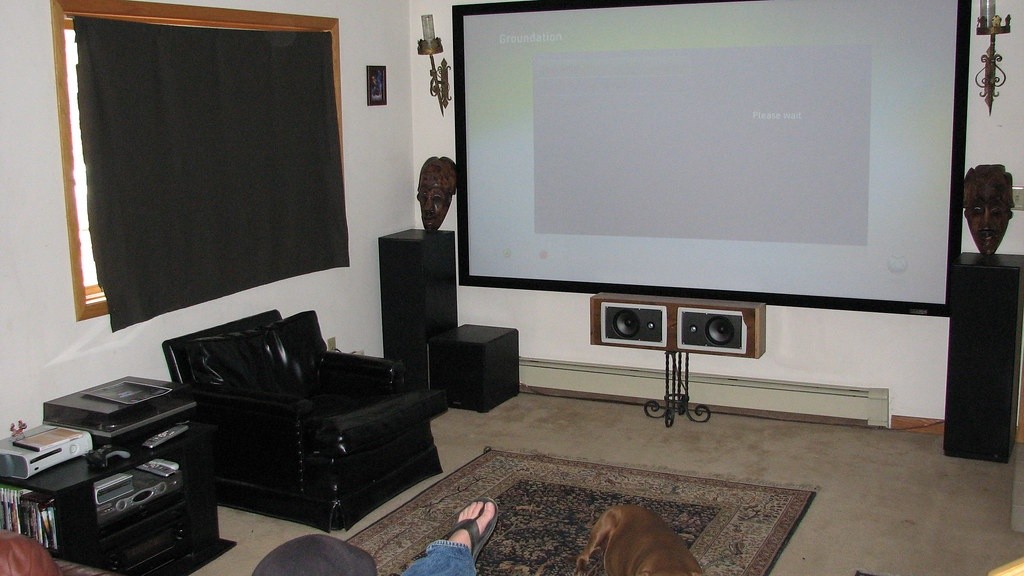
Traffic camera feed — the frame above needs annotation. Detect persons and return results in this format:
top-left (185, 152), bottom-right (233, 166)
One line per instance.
top-left (401, 496), bottom-right (498, 576)
top-left (416, 157), bottom-right (457, 233)
top-left (962, 164), bottom-right (1015, 256)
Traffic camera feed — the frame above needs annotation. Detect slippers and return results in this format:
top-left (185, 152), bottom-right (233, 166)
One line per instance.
top-left (446, 497), bottom-right (498, 563)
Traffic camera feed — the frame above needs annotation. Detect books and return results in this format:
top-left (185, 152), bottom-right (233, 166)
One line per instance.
top-left (0, 488), bottom-right (58, 551)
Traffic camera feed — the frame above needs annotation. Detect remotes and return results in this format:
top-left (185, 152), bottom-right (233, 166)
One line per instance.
top-left (142, 424), bottom-right (189, 450)
top-left (136, 459), bottom-right (175, 477)
top-left (148, 458), bottom-right (179, 470)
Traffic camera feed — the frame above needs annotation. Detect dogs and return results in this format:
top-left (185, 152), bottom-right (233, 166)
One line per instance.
top-left (575, 504), bottom-right (704, 576)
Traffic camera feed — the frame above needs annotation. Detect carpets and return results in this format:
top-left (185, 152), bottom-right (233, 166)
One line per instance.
top-left (344, 446), bottom-right (822, 576)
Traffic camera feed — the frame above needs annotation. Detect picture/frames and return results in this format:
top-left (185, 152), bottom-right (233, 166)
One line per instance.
top-left (366, 65), bottom-right (387, 106)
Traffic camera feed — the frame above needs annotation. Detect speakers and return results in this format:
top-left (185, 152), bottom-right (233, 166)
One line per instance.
top-left (589, 293), bottom-right (767, 359)
top-left (426, 325), bottom-right (520, 413)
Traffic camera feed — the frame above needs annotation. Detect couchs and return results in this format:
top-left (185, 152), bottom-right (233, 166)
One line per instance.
top-left (162, 309), bottom-right (446, 534)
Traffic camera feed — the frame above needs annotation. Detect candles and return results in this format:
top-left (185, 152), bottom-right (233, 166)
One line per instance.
top-left (979, 0), bottom-right (995, 28)
top-left (422, 14), bottom-right (435, 47)
